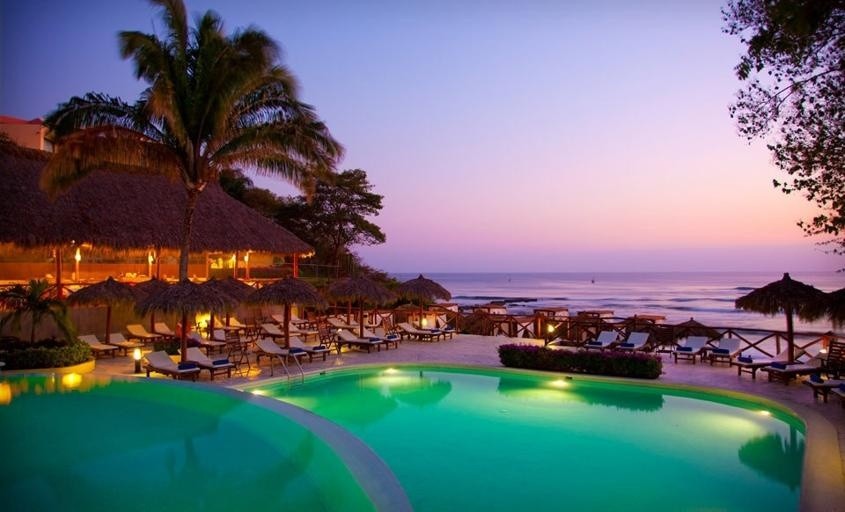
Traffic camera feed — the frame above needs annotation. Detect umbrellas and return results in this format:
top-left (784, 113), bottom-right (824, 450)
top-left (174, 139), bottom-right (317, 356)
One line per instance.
top-left (655, 317), bottom-right (723, 344)
top-left (735, 272), bottom-right (830, 363)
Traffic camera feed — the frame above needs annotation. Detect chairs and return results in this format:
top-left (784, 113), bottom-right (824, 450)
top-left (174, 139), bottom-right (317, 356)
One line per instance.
top-left (581, 326), bottom-right (845, 411)
top-left (75, 308), bottom-right (456, 382)
